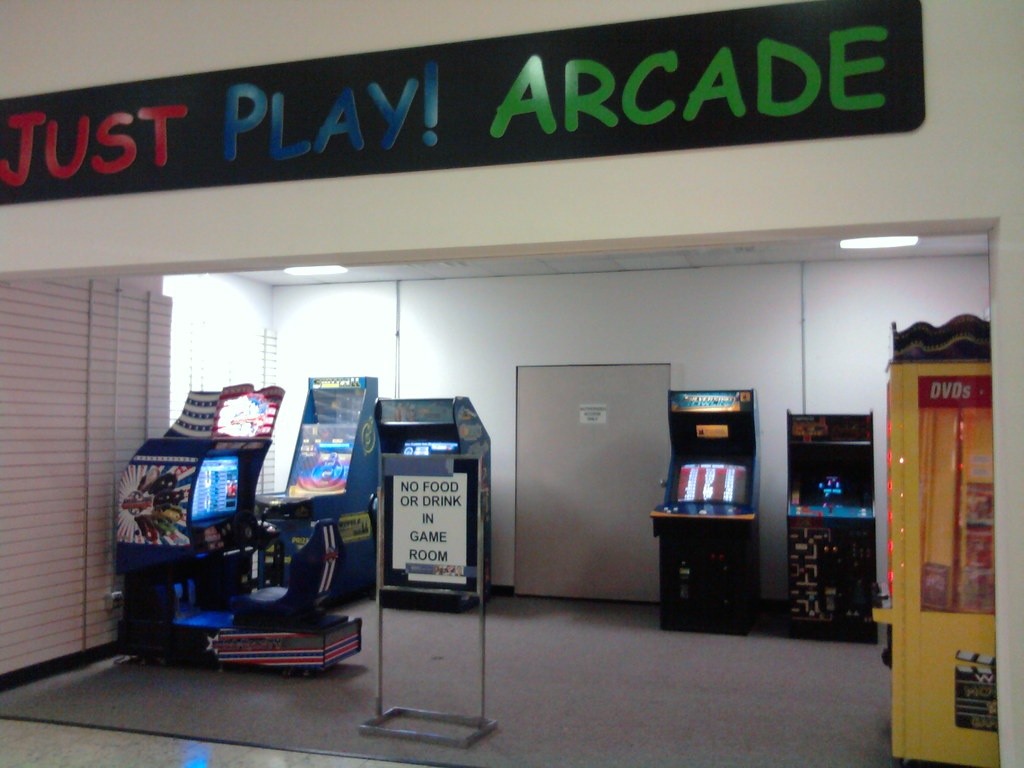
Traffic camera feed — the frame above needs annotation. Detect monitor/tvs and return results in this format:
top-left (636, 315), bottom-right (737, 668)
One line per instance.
top-left (190, 456), bottom-right (239, 521)
top-left (675, 461), bottom-right (747, 507)
top-left (800, 464), bottom-right (861, 507)
top-left (401, 438), bottom-right (460, 455)
